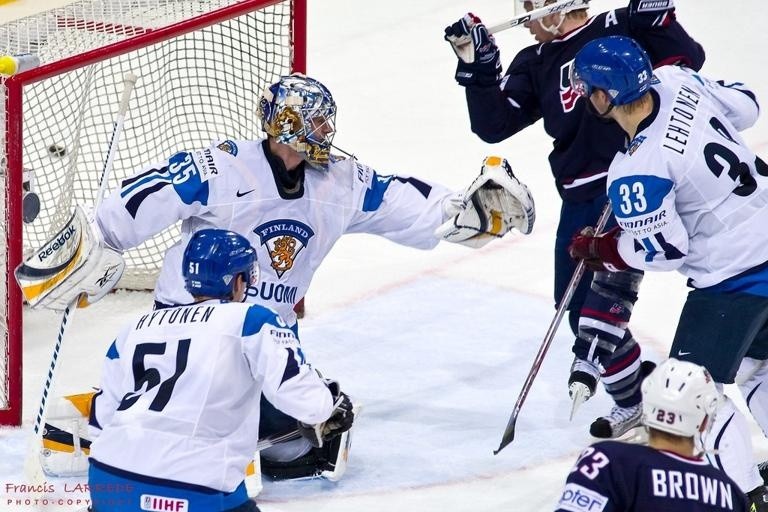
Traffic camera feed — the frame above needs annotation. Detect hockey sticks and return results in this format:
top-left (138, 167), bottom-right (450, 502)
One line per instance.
top-left (22, 73), bottom-right (139, 509)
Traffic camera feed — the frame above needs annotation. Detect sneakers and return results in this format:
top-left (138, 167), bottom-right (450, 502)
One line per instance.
top-left (570, 357), bottom-right (599, 397)
top-left (744, 462), bottom-right (767, 512)
top-left (591, 403), bottom-right (644, 440)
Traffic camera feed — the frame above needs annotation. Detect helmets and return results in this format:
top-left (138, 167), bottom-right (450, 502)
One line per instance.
top-left (257, 72), bottom-right (335, 174)
top-left (568, 35), bottom-right (653, 105)
top-left (181, 227), bottom-right (260, 300)
top-left (513, 0), bottom-right (591, 17)
top-left (641, 357), bottom-right (719, 451)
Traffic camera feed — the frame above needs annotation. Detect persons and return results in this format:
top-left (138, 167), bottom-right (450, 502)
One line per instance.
top-left (12, 72), bottom-right (535, 479)
top-left (548, 359), bottom-right (750, 512)
top-left (570, 35), bottom-right (768, 388)
top-left (443, 0), bottom-right (706, 440)
top-left (36, 230), bottom-right (353, 512)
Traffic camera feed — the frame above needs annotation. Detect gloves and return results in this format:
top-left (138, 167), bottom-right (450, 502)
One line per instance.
top-left (322, 391), bottom-right (354, 441)
top-left (444, 11), bottom-right (505, 86)
top-left (569, 227), bottom-right (644, 278)
